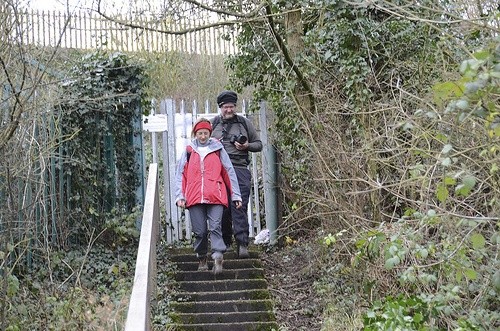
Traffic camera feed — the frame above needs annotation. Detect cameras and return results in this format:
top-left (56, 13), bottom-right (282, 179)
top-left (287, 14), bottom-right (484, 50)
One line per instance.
top-left (230, 134), bottom-right (247, 144)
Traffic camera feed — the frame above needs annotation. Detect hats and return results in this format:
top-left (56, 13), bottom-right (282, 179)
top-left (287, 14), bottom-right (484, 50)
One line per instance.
top-left (216, 91), bottom-right (238, 108)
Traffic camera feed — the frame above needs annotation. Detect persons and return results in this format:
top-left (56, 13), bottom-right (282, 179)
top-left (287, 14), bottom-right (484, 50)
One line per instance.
top-left (208, 89), bottom-right (262, 258)
top-left (177, 117), bottom-right (241, 275)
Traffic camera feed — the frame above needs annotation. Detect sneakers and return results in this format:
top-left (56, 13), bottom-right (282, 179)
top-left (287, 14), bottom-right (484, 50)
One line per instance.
top-left (212, 258), bottom-right (225, 275)
top-left (237, 243), bottom-right (249, 258)
top-left (197, 256), bottom-right (209, 271)
top-left (225, 244), bottom-right (234, 252)
